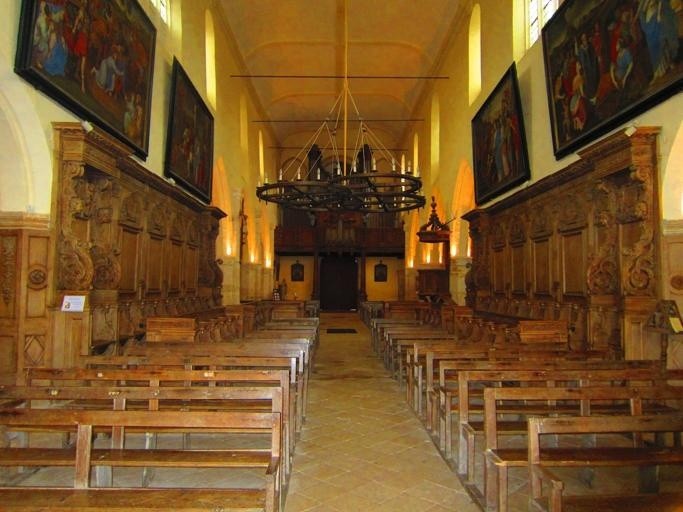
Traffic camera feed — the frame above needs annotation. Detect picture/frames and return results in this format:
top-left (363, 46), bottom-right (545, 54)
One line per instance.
top-left (163, 53), bottom-right (214, 205)
top-left (539, 0), bottom-right (682, 163)
top-left (469, 60), bottom-right (530, 206)
top-left (12, 0), bottom-right (157, 162)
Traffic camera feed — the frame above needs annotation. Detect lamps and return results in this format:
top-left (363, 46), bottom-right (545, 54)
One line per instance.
top-left (641, 299), bottom-right (682, 382)
top-left (255, 0), bottom-right (425, 210)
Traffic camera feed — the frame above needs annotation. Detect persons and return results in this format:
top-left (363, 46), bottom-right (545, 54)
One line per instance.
top-left (33, 2), bottom-right (149, 147)
top-left (553, 0), bottom-right (683, 140)
top-left (471, 99), bottom-right (526, 198)
